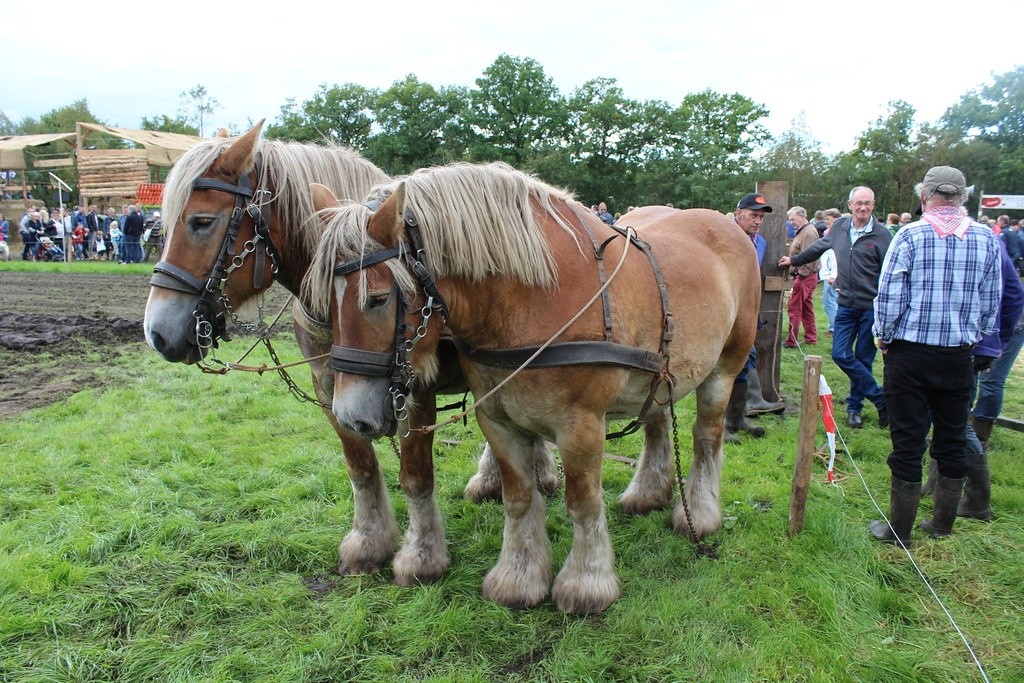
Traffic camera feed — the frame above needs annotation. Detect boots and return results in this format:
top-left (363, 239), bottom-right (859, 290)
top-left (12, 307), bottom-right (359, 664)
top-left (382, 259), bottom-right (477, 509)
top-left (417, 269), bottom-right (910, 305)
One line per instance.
top-left (723, 428), bottom-right (741, 445)
top-left (956, 452), bottom-right (992, 519)
top-left (870, 475), bottom-right (922, 550)
top-left (920, 458), bottom-right (938, 496)
top-left (724, 381), bottom-right (766, 438)
top-left (970, 414), bottom-right (993, 449)
top-left (918, 473), bottom-right (965, 539)
top-left (744, 369), bottom-right (785, 418)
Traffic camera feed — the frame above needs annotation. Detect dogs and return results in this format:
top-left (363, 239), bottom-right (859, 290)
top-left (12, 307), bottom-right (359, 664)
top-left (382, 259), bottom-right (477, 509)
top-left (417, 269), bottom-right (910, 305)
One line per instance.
top-left (0, 241), bottom-right (10, 263)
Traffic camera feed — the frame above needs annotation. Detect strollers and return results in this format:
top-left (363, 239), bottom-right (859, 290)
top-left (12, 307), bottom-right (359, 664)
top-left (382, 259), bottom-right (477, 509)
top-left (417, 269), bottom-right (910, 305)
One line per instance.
top-left (35, 232), bottom-right (64, 261)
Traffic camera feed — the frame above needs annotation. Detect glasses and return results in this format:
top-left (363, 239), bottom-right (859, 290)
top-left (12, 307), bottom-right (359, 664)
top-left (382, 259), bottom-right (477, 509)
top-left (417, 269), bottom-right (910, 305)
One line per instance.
top-left (852, 202), bottom-right (874, 207)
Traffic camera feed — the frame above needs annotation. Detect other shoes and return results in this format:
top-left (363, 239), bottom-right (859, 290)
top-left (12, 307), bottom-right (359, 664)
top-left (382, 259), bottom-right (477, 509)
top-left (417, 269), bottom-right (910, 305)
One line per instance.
top-left (783, 344), bottom-right (793, 349)
top-left (799, 341), bottom-right (810, 347)
top-left (824, 330), bottom-right (834, 338)
top-left (75, 254), bottom-right (113, 261)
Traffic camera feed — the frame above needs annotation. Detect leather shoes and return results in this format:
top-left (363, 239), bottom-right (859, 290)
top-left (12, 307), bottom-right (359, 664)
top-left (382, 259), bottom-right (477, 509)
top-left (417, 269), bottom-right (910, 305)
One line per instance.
top-left (878, 409), bottom-right (889, 429)
top-left (848, 412), bottom-right (862, 428)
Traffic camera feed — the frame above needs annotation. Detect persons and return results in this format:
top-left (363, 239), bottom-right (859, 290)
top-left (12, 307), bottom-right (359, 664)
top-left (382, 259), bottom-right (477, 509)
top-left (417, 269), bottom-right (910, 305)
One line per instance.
top-left (721, 192), bottom-right (851, 444)
top-left (0, 179), bottom-right (166, 265)
top-left (591, 201), bottom-right (673, 224)
top-left (776, 185), bottom-right (888, 429)
top-left (871, 166), bottom-right (1002, 547)
top-left (879, 213), bottom-right (1024, 520)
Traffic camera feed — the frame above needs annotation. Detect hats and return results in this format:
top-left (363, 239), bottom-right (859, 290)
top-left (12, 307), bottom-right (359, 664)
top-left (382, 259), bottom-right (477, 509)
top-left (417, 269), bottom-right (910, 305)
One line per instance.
top-left (737, 193), bottom-right (773, 213)
top-left (153, 211), bottom-right (161, 218)
top-left (923, 165), bottom-right (966, 195)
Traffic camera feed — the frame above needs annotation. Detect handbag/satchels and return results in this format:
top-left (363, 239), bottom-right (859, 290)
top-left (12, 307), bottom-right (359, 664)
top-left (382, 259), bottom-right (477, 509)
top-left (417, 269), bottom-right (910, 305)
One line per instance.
top-left (143, 229), bottom-right (152, 242)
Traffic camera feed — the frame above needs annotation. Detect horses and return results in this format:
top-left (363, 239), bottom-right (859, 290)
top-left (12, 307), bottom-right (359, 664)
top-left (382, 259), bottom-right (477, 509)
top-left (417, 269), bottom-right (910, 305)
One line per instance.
top-left (300, 160), bottom-right (763, 616)
top-left (143, 118), bottom-right (559, 591)
